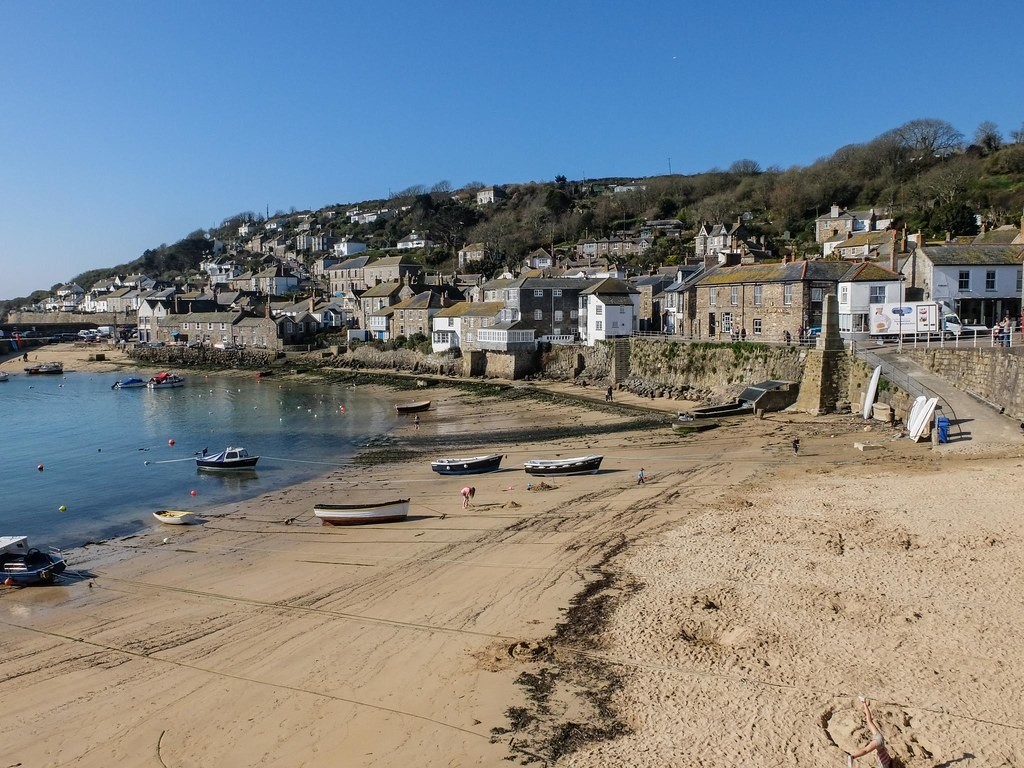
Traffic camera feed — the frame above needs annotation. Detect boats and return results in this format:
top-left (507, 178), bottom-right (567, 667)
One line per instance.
top-left (0, 372), bottom-right (9, 382)
top-left (524, 455), bottom-right (606, 477)
top-left (395, 400), bottom-right (432, 413)
top-left (907, 396), bottom-right (927, 432)
top-left (0, 535), bottom-right (67, 586)
top-left (112, 378), bottom-right (148, 389)
top-left (313, 498), bottom-right (412, 525)
top-left (863, 365), bottom-right (882, 419)
top-left (154, 509), bottom-right (195, 525)
top-left (196, 447), bottom-right (260, 470)
top-left (25, 361), bottom-right (64, 374)
top-left (431, 454), bottom-right (504, 476)
top-left (910, 396), bottom-right (940, 443)
top-left (147, 373), bottom-right (184, 389)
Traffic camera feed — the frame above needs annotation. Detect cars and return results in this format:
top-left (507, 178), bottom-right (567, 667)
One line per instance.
top-left (0, 329), bottom-right (109, 339)
top-left (806, 327), bottom-right (821, 347)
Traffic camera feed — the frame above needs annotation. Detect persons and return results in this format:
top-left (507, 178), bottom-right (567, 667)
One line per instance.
top-left (874, 305), bottom-right (892, 333)
top-left (637, 467), bottom-right (645, 485)
top-left (606, 385), bottom-right (613, 402)
top-left (460, 486), bottom-right (475, 508)
top-left (793, 438), bottom-right (800, 455)
top-left (993, 315), bottom-right (1011, 347)
top-left (729, 326), bottom-right (746, 344)
top-left (797, 325), bottom-right (804, 346)
top-left (661, 324), bottom-right (669, 341)
top-left (414, 413), bottom-right (420, 430)
top-left (783, 330), bottom-right (790, 346)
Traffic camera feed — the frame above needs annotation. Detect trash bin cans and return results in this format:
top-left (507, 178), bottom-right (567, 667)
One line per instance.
top-left (938, 417), bottom-right (949, 423)
top-left (938, 422), bottom-right (948, 442)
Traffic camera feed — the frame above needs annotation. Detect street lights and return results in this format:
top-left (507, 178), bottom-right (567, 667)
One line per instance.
top-left (898, 270), bottom-right (905, 348)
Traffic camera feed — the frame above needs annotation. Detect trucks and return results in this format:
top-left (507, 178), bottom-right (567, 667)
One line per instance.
top-left (869, 301), bottom-right (963, 344)
top-left (97, 326), bottom-right (115, 339)
